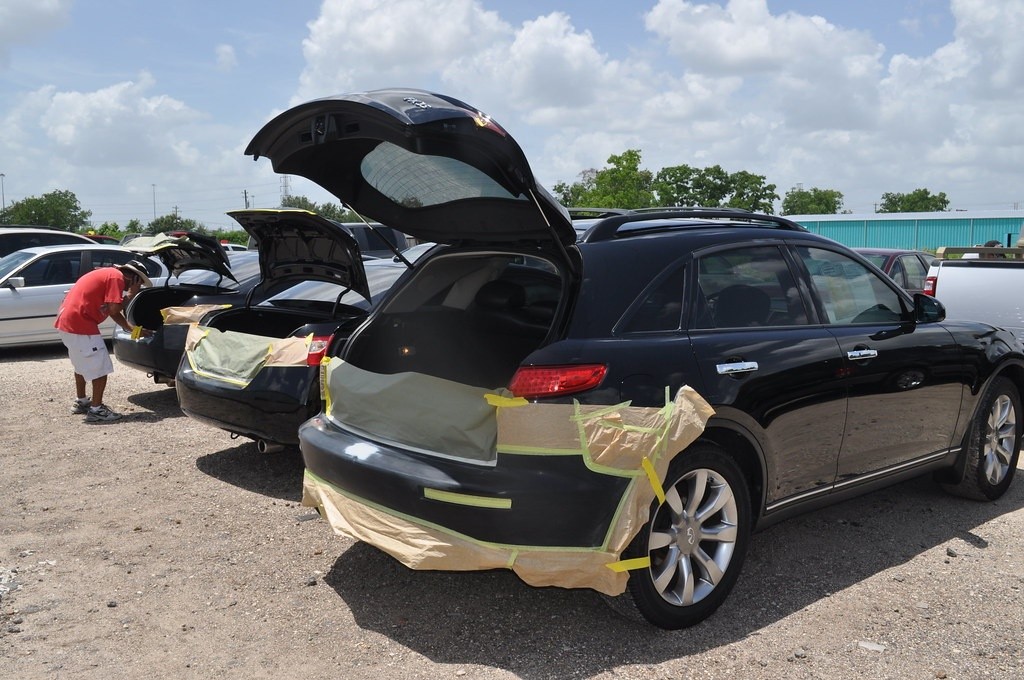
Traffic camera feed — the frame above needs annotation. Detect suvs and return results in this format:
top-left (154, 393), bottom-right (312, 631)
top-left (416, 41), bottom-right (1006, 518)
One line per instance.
top-left (0, 224), bottom-right (101, 282)
top-left (243, 87), bottom-right (1023, 629)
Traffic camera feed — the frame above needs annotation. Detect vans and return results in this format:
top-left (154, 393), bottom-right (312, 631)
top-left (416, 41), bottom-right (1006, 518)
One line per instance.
top-left (247, 223), bottom-right (409, 259)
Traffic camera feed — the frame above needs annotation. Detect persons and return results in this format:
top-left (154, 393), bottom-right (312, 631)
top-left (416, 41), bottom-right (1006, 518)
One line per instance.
top-left (54, 260), bottom-right (155, 421)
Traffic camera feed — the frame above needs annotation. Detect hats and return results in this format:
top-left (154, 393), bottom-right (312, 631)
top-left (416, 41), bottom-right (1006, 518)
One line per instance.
top-left (114, 259), bottom-right (153, 286)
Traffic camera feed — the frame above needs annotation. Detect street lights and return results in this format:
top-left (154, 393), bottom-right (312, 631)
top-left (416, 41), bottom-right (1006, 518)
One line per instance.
top-left (152, 184), bottom-right (156, 219)
top-left (0, 174), bottom-right (5, 210)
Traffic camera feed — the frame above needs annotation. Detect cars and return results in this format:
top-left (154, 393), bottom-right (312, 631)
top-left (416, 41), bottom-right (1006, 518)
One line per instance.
top-left (0, 244), bottom-right (176, 348)
top-left (174, 207), bottom-right (437, 454)
top-left (731, 247), bottom-right (937, 325)
top-left (112, 229), bottom-right (261, 387)
top-left (699, 255), bottom-right (764, 297)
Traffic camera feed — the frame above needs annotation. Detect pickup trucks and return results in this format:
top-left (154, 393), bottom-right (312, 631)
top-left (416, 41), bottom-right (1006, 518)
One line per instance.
top-left (923, 247), bottom-right (1024, 343)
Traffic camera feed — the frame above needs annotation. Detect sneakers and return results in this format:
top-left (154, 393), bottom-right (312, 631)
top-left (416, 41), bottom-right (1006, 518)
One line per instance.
top-left (71, 400), bottom-right (93, 414)
top-left (87, 404), bottom-right (122, 422)
